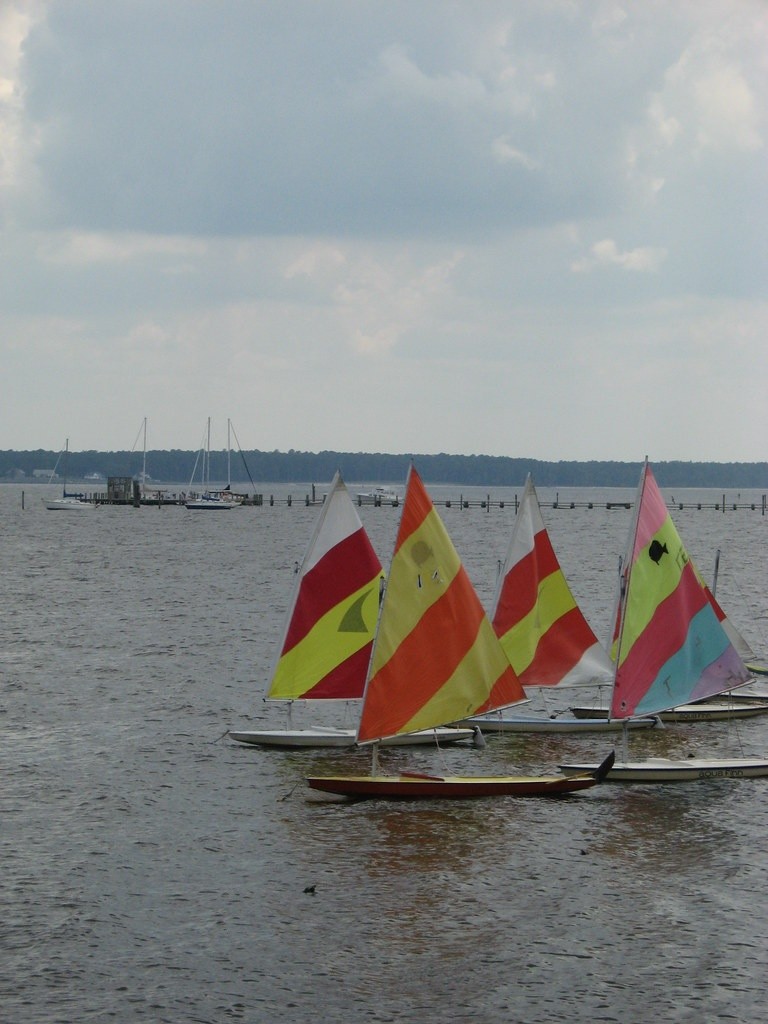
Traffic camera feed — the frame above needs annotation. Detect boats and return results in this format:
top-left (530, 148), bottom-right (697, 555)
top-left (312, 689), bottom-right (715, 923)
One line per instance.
top-left (355, 486), bottom-right (406, 503)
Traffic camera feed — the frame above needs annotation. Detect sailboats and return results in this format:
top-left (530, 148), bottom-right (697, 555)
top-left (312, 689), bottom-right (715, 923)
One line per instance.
top-left (555, 451), bottom-right (767, 782)
top-left (40, 438), bottom-right (102, 510)
top-left (183, 416), bottom-right (257, 510)
top-left (302, 456), bottom-right (616, 801)
top-left (123, 417), bottom-right (155, 500)
top-left (226, 468), bottom-right (488, 747)
top-left (441, 468), bottom-right (658, 732)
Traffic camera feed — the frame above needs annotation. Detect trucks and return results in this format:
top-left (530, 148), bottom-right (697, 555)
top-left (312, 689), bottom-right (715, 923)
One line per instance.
top-left (33, 469), bottom-right (59, 478)
